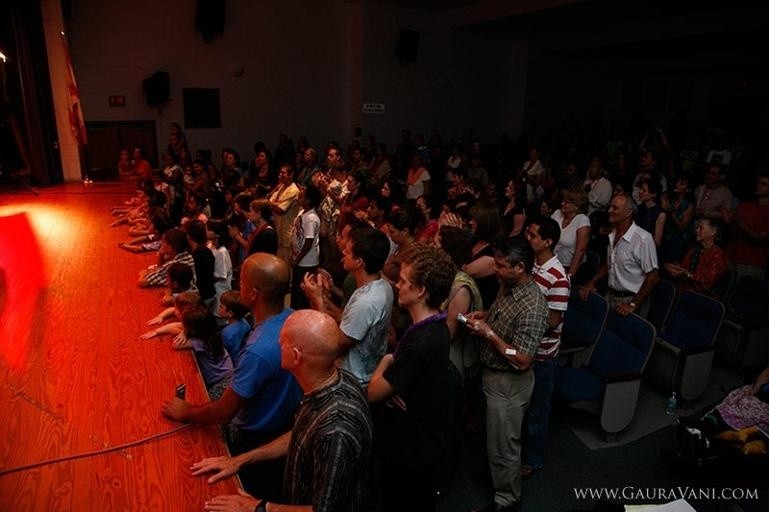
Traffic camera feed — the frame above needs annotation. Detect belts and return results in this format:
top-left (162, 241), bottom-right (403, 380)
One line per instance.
top-left (607, 286), bottom-right (641, 298)
top-left (482, 364), bottom-right (533, 375)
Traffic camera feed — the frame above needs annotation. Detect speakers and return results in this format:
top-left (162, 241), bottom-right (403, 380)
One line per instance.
top-left (396, 28), bottom-right (420, 63)
top-left (195, 0), bottom-right (226, 41)
top-left (141, 70), bottom-right (169, 105)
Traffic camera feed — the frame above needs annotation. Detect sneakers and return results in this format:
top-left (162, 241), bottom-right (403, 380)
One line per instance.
top-left (519, 461), bottom-right (547, 474)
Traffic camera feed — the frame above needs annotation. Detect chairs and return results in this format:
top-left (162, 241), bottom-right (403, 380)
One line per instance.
top-left (723, 274), bottom-right (764, 329)
top-left (706, 266), bottom-right (734, 305)
top-left (637, 281), bottom-right (677, 334)
top-left (550, 307), bottom-right (657, 405)
top-left (557, 282), bottom-right (610, 354)
top-left (654, 289), bottom-right (726, 399)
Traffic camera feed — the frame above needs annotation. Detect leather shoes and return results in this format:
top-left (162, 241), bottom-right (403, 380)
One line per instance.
top-left (479, 496), bottom-right (524, 512)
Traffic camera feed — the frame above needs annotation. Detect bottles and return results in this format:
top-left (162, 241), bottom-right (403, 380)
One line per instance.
top-left (666, 390), bottom-right (678, 417)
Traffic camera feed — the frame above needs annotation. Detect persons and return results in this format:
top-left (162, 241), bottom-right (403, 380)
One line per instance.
top-left (110, 122), bottom-right (768, 511)
